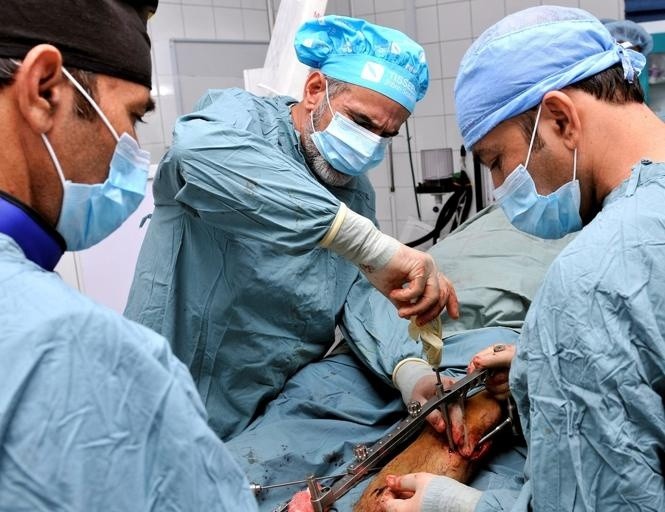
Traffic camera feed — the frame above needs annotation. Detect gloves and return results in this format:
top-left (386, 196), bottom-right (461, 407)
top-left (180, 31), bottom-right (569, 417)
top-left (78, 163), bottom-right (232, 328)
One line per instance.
top-left (468, 343), bottom-right (516, 401)
top-left (392, 357), bottom-right (475, 457)
top-left (380, 472), bottom-right (483, 512)
top-left (318, 200), bottom-right (460, 327)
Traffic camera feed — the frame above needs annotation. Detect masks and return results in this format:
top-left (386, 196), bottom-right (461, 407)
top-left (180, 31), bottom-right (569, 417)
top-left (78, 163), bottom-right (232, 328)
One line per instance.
top-left (311, 79), bottom-right (393, 177)
top-left (491, 104), bottom-right (593, 240)
top-left (41, 65), bottom-right (152, 251)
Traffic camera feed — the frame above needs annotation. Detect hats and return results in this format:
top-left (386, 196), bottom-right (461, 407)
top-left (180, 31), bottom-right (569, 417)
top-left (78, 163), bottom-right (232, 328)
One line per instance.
top-left (1, 0), bottom-right (157, 90)
top-left (293, 15), bottom-right (429, 114)
top-left (454, 4), bottom-right (647, 151)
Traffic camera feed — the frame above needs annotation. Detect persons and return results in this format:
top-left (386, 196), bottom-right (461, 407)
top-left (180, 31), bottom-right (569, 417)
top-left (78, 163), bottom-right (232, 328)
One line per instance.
top-left (122, 13), bottom-right (460, 442)
top-left (380, 5), bottom-right (665, 512)
top-left (605, 18), bottom-right (652, 104)
top-left (0, 0), bottom-right (260, 512)
top-left (351, 388), bottom-right (501, 512)
